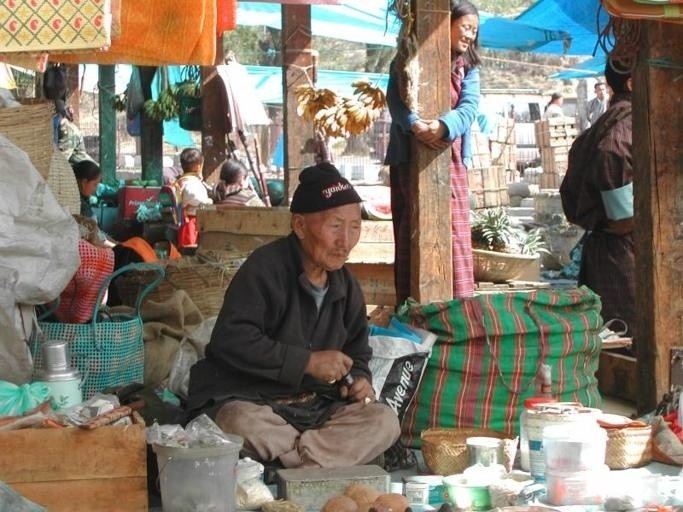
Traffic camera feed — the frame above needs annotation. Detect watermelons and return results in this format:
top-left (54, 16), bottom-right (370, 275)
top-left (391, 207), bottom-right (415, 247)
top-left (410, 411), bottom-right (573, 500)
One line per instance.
top-left (249, 176), bottom-right (285, 206)
top-left (361, 199), bottom-right (393, 221)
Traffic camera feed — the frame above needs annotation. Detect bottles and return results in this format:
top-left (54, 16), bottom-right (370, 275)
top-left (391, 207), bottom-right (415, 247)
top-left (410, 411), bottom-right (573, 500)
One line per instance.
top-left (519, 396), bottom-right (557, 472)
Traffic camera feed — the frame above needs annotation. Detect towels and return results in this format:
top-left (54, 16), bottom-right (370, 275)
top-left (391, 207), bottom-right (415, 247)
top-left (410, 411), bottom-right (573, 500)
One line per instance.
top-left (0, 0), bottom-right (235, 74)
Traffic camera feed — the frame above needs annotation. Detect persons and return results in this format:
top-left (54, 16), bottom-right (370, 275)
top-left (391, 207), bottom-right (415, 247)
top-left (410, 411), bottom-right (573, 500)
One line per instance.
top-left (161, 147), bottom-right (212, 249)
top-left (560, 48), bottom-right (637, 358)
top-left (586, 82), bottom-right (611, 130)
top-left (382, 0), bottom-right (482, 303)
top-left (52, 98), bottom-right (92, 164)
top-left (208, 160), bottom-right (267, 208)
top-left (542, 92), bottom-right (564, 118)
top-left (76, 157), bottom-right (119, 251)
top-left (185, 160), bottom-right (403, 485)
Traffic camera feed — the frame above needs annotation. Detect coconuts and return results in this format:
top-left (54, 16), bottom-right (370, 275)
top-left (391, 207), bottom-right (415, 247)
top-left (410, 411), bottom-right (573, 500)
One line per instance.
top-left (321, 486), bottom-right (409, 512)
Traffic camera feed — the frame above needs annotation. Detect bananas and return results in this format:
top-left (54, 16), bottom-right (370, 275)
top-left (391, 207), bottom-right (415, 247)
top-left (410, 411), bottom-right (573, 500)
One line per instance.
top-left (143, 79), bottom-right (200, 123)
top-left (294, 79), bottom-right (387, 140)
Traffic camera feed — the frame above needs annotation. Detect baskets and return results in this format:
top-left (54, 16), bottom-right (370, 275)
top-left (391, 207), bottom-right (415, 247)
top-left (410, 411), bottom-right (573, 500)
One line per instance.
top-left (472, 249), bottom-right (540, 282)
top-left (421, 427), bottom-right (519, 477)
top-left (605, 426), bottom-right (653, 470)
top-left (0, 100), bottom-right (81, 216)
top-left (114, 249), bottom-right (253, 318)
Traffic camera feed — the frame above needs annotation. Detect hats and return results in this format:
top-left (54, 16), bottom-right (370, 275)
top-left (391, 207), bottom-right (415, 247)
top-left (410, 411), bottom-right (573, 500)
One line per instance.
top-left (290, 161), bottom-right (363, 213)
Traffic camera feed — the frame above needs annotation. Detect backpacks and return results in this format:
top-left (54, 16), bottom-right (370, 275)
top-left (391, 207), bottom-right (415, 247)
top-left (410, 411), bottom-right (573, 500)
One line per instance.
top-left (158, 174), bottom-right (196, 230)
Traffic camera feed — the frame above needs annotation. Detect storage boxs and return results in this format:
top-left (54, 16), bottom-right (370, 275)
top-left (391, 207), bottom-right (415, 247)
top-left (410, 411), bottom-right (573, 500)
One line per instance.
top-left (0, 411), bottom-right (149, 512)
top-left (342, 221), bottom-right (395, 306)
top-left (195, 207), bottom-right (292, 251)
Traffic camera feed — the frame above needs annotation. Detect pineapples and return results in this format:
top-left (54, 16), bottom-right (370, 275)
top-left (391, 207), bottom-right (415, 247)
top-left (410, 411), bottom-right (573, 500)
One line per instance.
top-left (468, 206), bottom-right (553, 273)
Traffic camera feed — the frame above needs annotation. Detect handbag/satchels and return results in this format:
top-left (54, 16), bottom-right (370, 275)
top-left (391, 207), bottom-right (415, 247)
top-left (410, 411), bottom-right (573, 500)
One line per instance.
top-left (368, 285), bottom-right (604, 450)
top-left (31, 238), bottom-right (165, 402)
top-left (179, 83), bottom-right (203, 131)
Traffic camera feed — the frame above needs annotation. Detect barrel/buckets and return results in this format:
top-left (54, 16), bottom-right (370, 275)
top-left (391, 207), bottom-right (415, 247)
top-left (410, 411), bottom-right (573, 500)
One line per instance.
top-left (524, 408), bottom-right (603, 485)
top-left (151, 431), bottom-right (245, 512)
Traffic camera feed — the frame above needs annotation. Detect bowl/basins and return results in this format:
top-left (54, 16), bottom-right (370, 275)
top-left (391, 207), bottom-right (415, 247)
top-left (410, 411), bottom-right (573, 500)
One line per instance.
top-left (443, 473), bottom-right (494, 511)
